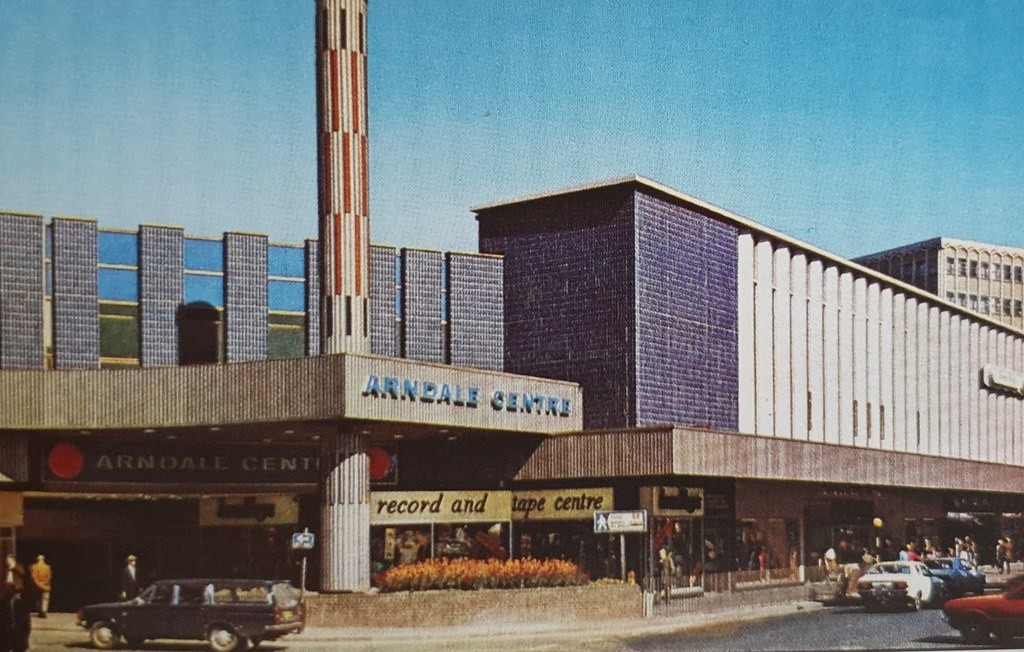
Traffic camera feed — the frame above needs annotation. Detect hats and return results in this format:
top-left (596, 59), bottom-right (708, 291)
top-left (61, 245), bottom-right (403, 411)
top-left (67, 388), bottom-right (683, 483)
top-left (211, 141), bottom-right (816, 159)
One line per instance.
top-left (127, 554), bottom-right (137, 560)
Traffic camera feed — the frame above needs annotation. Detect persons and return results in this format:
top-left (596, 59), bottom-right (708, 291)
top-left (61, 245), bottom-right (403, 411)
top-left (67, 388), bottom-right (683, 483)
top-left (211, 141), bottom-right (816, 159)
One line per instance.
top-left (120, 555), bottom-right (148, 600)
top-left (1, 584), bottom-right (30, 652)
top-left (882, 535), bottom-right (1013, 576)
top-left (2, 554), bottom-right (25, 595)
top-left (30, 553), bottom-right (54, 618)
top-left (157, 586), bottom-right (170, 601)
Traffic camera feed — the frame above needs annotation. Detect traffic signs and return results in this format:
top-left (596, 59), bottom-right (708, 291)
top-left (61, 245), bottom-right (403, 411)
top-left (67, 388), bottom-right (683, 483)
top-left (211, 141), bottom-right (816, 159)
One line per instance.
top-left (292, 534), bottom-right (315, 548)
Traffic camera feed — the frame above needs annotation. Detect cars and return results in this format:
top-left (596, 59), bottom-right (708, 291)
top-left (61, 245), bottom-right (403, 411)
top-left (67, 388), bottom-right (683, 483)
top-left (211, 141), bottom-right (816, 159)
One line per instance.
top-left (857, 561), bottom-right (948, 612)
top-left (941, 573), bottom-right (1024, 644)
top-left (75, 576), bottom-right (305, 652)
top-left (928, 557), bottom-right (986, 596)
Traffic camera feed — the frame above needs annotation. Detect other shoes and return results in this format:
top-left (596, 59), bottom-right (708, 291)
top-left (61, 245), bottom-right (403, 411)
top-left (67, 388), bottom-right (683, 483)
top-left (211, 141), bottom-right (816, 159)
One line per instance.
top-left (846, 590), bottom-right (862, 598)
top-left (38, 611), bottom-right (47, 617)
